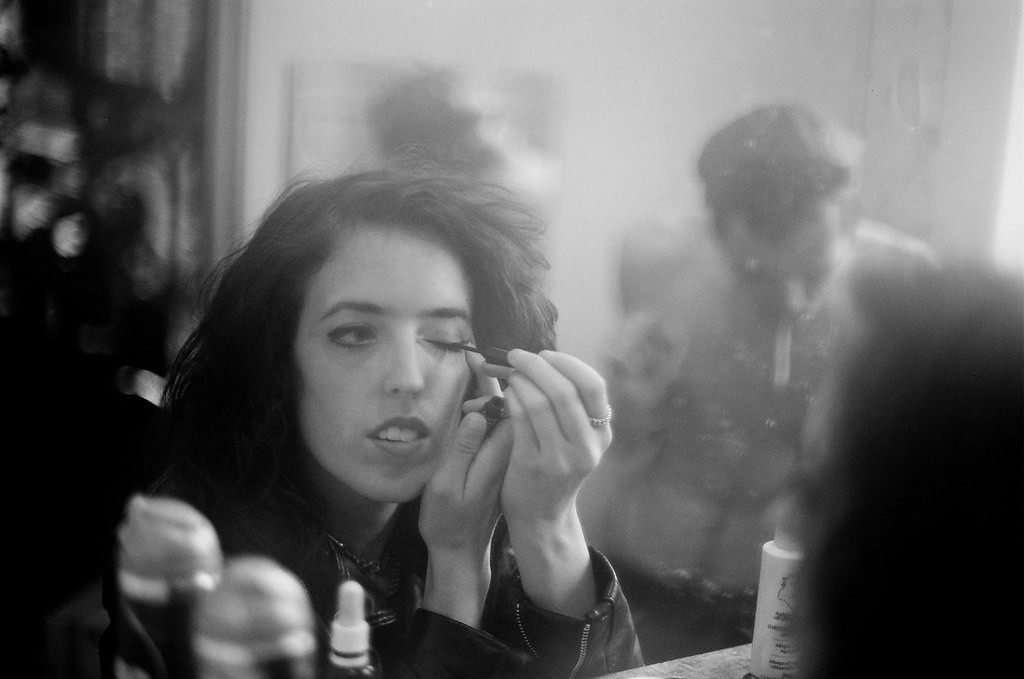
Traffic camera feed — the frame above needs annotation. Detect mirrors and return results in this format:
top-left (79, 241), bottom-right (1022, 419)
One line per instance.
top-left (240, 4), bottom-right (1010, 593)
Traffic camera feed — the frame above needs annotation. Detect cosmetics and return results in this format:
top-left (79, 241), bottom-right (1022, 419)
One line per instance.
top-left (445, 342), bottom-right (518, 436)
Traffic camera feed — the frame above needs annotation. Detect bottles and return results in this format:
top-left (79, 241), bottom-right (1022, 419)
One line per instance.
top-left (748, 507), bottom-right (810, 678)
top-left (328, 580), bottom-right (382, 679)
top-left (193, 557), bottom-right (315, 678)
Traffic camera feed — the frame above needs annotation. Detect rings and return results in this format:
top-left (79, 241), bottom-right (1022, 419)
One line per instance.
top-left (586, 404), bottom-right (613, 427)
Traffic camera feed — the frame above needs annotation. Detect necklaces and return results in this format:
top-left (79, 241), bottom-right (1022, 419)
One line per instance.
top-left (326, 529), bottom-right (399, 630)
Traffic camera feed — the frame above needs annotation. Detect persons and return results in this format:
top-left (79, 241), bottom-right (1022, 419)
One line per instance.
top-left (657, 94), bottom-right (944, 542)
top-left (100, 140), bottom-right (647, 679)
top-left (774, 260), bottom-right (1024, 679)
